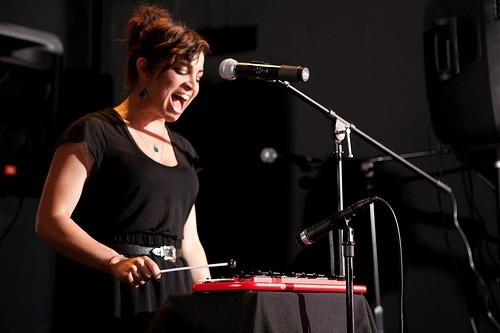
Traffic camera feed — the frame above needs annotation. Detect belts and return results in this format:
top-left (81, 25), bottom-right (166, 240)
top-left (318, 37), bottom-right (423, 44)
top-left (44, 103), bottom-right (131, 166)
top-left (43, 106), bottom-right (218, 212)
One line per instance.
top-left (103, 243), bottom-right (183, 264)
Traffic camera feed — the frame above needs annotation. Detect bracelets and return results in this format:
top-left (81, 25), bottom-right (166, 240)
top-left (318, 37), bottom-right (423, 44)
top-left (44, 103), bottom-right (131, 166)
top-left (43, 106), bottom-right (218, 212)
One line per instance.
top-left (107, 254), bottom-right (124, 274)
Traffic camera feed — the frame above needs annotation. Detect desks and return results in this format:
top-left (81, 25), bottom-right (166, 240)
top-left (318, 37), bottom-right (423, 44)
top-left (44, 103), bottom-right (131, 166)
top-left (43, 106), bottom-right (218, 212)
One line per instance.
top-left (148, 291), bottom-right (378, 333)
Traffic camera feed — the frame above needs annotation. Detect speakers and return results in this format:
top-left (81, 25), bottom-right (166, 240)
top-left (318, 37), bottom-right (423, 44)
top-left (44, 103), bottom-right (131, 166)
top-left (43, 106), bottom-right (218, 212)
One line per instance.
top-left (1, 58), bottom-right (116, 198)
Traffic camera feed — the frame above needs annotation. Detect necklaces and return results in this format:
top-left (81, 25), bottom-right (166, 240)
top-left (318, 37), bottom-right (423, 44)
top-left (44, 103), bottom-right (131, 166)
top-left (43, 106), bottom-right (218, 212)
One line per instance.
top-left (122, 102), bottom-right (165, 153)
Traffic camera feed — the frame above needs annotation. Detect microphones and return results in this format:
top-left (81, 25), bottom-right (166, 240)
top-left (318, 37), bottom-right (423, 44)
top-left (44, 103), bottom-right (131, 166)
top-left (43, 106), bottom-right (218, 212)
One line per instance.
top-left (219, 58), bottom-right (309, 82)
top-left (296, 198), bottom-right (375, 249)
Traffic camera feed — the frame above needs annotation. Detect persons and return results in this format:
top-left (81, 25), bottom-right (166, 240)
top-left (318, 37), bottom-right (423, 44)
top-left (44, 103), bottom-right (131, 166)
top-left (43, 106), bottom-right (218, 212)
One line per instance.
top-left (34, 6), bottom-right (213, 333)
